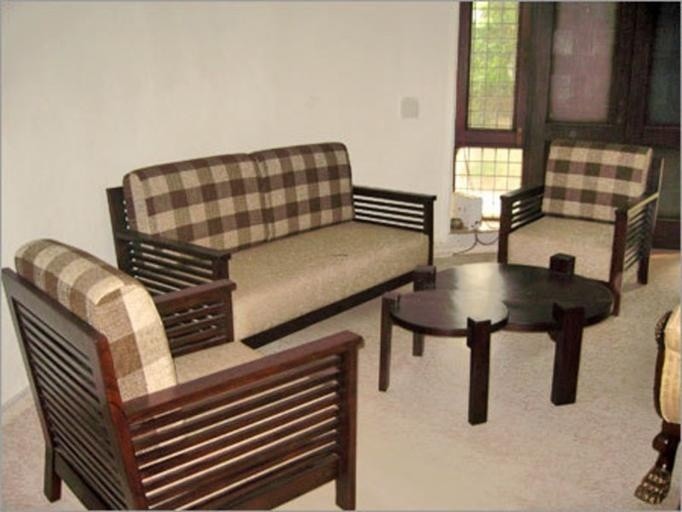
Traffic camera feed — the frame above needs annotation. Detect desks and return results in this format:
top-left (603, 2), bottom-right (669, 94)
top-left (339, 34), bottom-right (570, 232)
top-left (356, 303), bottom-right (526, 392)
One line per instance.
top-left (378, 254), bottom-right (613, 425)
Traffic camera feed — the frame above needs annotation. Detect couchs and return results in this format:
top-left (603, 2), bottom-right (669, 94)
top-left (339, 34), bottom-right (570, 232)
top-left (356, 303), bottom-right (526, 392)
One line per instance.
top-left (107, 143), bottom-right (438, 349)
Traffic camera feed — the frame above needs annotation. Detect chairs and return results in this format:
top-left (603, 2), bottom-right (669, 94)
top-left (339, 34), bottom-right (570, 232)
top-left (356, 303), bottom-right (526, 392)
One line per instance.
top-left (632, 306), bottom-right (682, 504)
top-left (498, 138), bottom-right (665, 317)
top-left (2, 236), bottom-right (365, 511)
